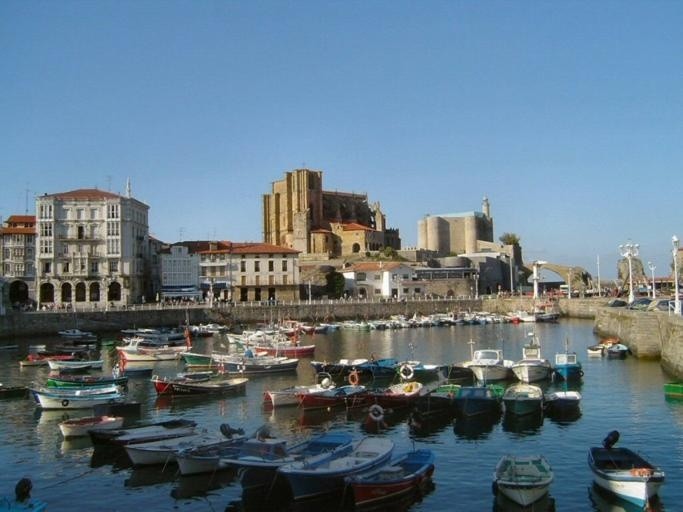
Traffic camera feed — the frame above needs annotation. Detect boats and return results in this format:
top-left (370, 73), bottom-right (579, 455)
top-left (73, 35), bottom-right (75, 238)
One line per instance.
top-left (510, 324), bottom-right (554, 384)
top-left (85, 413), bottom-right (196, 448)
top-left (219, 428), bottom-right (352, 492)
top-left (542, 376), bottom-right (584, 417)
top-left (0, 306), bottom-right (586, 418)
top-left (341, 448), bottom-right (437, 508)
top-left (276, 435), bottom-right (397, 505)
top-left (264, 371), bottom-right (338, 410)
top-left (293, 380), bottom-right (367, 411)
top-left (464, 347), bottom-right (515, 383)
top-left (346, 354), bottom-right (398, 388)
top-left (109, 424), bottom-right (207, 448)
top-left (588, 432), bottom-right (669, 505)
top-left (120, 423), bottom-right (250, 466)
top-left (587, 336), bottom-right (630, 360)
top-left (415, 383), bottom-right (461, 412)
top-left (493, 454), bottom-right (553, 505)
top-left (366, 382), bottom-right (423, 410)
top-left (438, 360), bottom-right (474, 382)
top-left (501, 377), bottom-right (544, 417)
top-left (451, 384), bottom-right (505, 418)
top-left (396, 343), bottom-right (441, 382)
top-left (57, 414), bottom-right (125, 438)
top-left (552, 336), bottom-right (585, 384)
top-left (174, 423), bottom-right (276, 476)
top-left (308, 356), bottom-right (370, 384)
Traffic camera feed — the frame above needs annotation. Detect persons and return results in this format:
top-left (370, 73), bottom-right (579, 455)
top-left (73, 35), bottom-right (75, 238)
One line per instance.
top-left (13, 295), bottom-right (274, 311)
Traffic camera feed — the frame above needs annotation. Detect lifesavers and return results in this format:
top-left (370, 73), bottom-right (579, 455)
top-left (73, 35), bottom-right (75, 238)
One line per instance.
top-left (369, 405), bottom-right (384, 421)
top-left (400, 364), bottom-right (414, 380)
top-left (348, 373), bottom-right (358, 385)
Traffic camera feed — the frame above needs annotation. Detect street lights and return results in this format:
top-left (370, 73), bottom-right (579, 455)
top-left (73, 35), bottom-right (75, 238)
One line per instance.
top-left (618, 242), bottom-right (639, 305)
top-left (647, 261), bottom-right (657, 300)
top-left (670, 235), bottom-right (681, 314)
